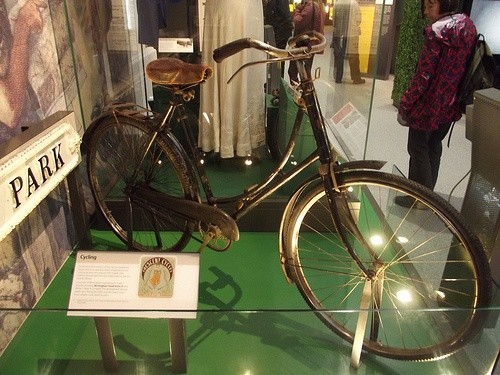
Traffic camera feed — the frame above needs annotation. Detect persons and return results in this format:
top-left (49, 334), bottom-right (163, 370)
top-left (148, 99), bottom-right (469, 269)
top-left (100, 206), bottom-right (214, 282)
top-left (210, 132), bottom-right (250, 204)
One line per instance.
top-left (265, 0), bottom-right (293, 79)
top-left (332, 0), bottom-right (365, 85)
top-left (291, 0), bottom-right (326, 90)
top-left (393, 0), bottom-right (477, 210)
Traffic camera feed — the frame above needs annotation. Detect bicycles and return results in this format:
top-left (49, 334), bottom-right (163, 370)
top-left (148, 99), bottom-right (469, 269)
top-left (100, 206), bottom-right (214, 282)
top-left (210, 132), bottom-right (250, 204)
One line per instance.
top-left (79, 30), bottom-right (493, 371)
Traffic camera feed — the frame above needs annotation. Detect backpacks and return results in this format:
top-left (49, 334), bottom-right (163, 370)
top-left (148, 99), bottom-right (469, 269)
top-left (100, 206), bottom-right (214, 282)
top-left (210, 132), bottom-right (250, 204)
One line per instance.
top-left (456, 33), bottom-right (494, 114)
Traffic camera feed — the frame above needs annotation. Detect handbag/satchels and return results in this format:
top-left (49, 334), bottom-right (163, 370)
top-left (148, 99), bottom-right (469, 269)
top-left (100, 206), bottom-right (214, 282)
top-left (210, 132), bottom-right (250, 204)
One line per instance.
top-left (293, 30), bottom-right (312, 48)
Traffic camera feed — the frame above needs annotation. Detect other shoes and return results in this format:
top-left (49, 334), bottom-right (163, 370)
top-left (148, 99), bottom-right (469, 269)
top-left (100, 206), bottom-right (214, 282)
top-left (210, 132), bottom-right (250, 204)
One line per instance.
top-left (394, 196), bottom-right (429, 210)
top-left (352, 78), bottom-right (366, 84)
top-left (335, 79), bottom-right (342, 83)
